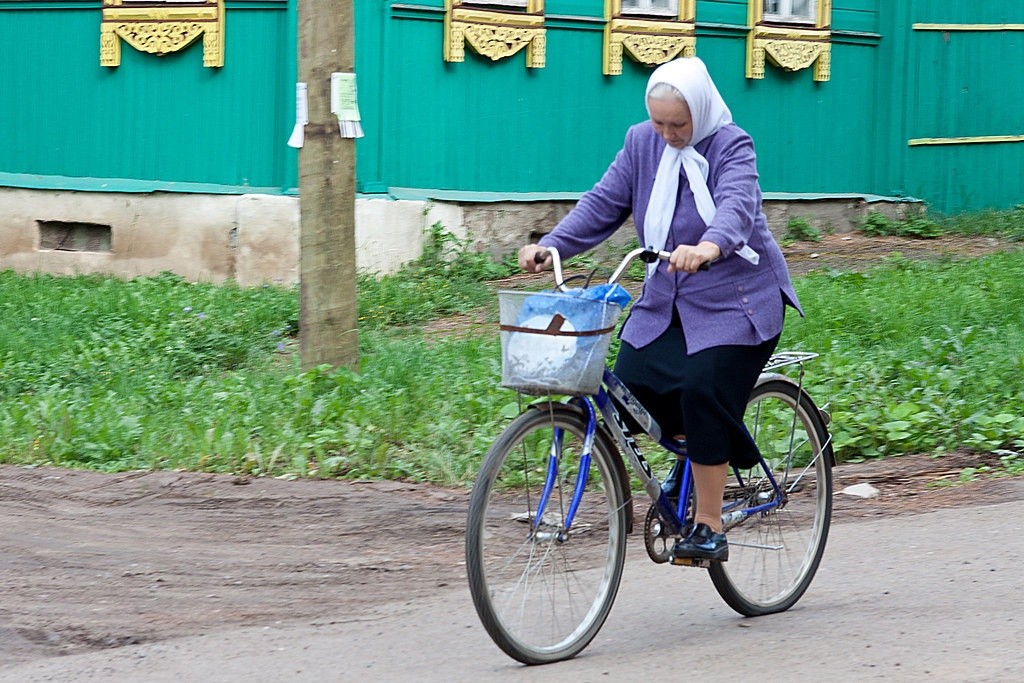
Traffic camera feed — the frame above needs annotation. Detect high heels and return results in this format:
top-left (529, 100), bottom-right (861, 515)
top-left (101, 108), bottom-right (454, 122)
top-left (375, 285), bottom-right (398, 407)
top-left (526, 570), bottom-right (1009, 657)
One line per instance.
top-left (660, 460), bottom-right (687, 497)
top-left (675, 523), bottom-right (729, 561)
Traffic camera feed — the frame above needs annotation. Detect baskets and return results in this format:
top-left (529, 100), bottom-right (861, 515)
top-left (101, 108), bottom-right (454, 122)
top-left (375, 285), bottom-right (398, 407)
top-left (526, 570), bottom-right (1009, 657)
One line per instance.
top-left (496, 288), bottom-right (623, 396)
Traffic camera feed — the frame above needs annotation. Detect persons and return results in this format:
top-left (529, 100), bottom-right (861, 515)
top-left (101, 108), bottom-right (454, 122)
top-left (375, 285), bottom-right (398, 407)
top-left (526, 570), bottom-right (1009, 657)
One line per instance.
top-left (518, 57), bottom-right (804, 561)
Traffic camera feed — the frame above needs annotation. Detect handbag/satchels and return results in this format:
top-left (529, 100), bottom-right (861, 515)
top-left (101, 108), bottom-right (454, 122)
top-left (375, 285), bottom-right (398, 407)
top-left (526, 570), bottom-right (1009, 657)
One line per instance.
top-left (506, 268), bottom-right (633, 396)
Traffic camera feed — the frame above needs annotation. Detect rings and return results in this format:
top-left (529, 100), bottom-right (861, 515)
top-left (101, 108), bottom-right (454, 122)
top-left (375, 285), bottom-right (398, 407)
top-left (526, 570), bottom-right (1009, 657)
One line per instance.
top-left (689, 267), bottom-right (695, 271)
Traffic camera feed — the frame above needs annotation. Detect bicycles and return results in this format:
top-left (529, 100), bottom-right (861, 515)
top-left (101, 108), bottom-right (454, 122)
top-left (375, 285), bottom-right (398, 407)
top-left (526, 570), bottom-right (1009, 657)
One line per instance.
top-left (466, 247), bottom-right (837, 665)
